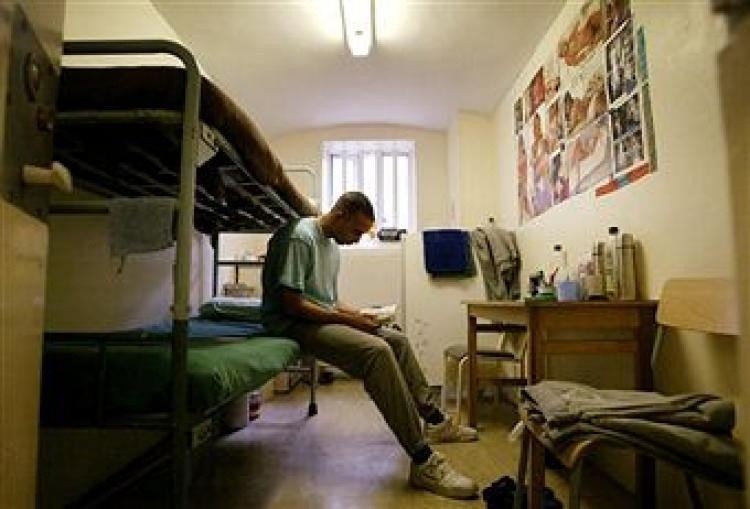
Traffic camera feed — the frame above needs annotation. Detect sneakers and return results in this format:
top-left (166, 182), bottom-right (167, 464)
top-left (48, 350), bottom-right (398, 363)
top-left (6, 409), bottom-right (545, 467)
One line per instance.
top-left (421, 408), bottom-right (477, 443)
top-left (409, 451), bottom-right (480, 499)
top-left (483, 474), bottom-right (563, 509)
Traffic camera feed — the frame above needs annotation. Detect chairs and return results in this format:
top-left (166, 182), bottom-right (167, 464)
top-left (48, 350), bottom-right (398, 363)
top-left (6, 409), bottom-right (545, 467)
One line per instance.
top-left (440, 299), bottom-right (529, 425)
top-left (513, 272), bottom-right (748, 508)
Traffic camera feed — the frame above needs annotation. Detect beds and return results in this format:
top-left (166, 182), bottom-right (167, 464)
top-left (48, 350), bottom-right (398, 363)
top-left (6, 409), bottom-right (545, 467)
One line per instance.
top-left (37, 295), bottom-right (320, 509)
top-left (36, 37), bottom-right (323, 316)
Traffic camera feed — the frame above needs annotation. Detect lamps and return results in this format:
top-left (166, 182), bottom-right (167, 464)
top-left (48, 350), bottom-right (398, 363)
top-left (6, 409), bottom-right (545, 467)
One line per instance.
top-left (339, 0), bottom-right (376, 58)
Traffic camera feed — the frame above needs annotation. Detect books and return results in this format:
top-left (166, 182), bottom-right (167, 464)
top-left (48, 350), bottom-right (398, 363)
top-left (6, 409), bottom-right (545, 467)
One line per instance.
top-left (353, 305), bottom-right (397, 325)
top-left (582, 232), bottom-right (639, 300)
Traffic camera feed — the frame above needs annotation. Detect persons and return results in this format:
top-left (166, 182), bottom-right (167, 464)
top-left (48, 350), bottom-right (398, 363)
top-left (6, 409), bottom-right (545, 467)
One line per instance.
top-left (262, 191), bottom-right (480, 499)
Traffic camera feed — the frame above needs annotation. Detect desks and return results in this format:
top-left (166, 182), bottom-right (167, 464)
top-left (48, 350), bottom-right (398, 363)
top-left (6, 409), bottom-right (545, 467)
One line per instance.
top-left (459, 295), bottom-right (663, 507)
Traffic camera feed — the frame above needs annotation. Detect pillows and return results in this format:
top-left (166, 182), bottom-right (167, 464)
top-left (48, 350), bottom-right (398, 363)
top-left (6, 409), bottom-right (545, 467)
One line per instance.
top-left (195, 294), bottom-right (266, 323)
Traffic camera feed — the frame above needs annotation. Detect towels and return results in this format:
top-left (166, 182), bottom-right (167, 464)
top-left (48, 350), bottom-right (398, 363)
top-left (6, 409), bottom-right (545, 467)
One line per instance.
top-left (104, 193), bottom-right (175, 276)
